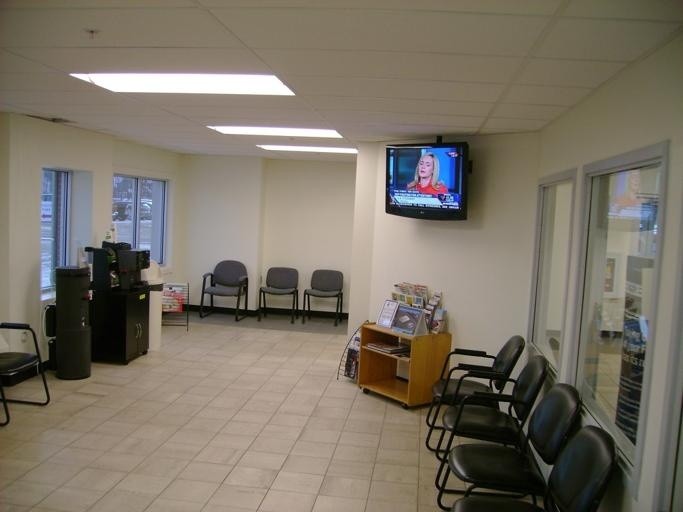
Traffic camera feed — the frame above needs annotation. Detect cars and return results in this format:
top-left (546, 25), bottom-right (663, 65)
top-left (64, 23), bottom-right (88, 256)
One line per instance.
top-left (109, 197), bottom-right (153, 223)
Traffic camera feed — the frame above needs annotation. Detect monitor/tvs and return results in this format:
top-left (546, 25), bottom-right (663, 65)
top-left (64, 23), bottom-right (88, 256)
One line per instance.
top-left (386, 142), bottom-right (469, 221)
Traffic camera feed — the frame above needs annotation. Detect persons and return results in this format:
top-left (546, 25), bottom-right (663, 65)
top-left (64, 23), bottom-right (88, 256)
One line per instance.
top-left (405, 152), bottom-right (448, 199)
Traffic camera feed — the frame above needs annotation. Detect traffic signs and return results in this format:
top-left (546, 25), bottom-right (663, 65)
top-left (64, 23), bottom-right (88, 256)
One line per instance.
top-left (40, 205), bottom-right (53, 222)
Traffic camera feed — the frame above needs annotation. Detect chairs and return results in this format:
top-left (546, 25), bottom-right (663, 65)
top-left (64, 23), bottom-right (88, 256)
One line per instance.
top-left (0, 322), bottom-right (50, 426)
top-left (425, 334), bottom-right (617, 511)
top-left (198, 260), bottom-right (343, 327)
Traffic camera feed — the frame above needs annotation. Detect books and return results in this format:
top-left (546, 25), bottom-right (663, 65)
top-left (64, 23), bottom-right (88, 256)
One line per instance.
top-left (365, 340), bottom-right (409, 354)
top-left (343, 332), bottom-right (361, 380)
top-left (389, 280), bottom-right (447, 334)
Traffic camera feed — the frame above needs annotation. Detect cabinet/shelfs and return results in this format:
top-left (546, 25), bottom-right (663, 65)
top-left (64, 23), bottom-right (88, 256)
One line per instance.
top-left (356, 322), bottom-right (452, 409)
top-left (90, 285), bottom-right (150, 364)
